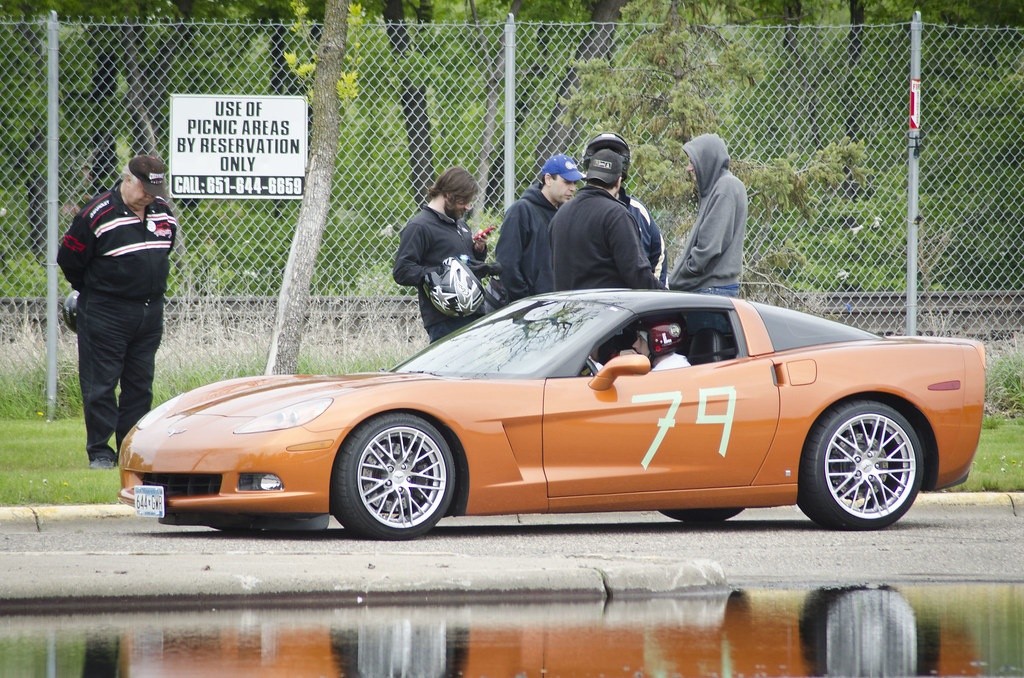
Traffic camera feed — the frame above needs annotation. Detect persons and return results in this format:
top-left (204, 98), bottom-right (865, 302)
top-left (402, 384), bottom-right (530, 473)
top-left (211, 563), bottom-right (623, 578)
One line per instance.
top-left (56, 155), bottom-right (178, 471)
top-left (392, 168), bottom-right (491, 345)
top-left (495, 155), bottom-right (583, 299)
top-left (588, 315), bottom-right (692, 376)
top-left (668, 133), bottom-right (743, 298)
top-left (547, 131), bottom-right (669, 292)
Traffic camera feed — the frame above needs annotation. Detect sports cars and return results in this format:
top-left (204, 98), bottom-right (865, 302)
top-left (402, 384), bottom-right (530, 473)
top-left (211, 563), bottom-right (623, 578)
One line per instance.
top-left (80, 584), bottom-right (979, 678)
top-left (118, 286), bottom-right (987, 541)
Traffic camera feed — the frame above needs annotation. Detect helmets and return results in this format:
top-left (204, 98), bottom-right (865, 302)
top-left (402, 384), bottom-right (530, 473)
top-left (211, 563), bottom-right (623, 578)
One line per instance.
top-left (424, 254), bottom-right (485, 318)
top-left (61, 291), bottom-right (77, 334)
top-left (584, 132), bottom-right (633, 181)
top-left (634, 311), bottom-right (683, 355)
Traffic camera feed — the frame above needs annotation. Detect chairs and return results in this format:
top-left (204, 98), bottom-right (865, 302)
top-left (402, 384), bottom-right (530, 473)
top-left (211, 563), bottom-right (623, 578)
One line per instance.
top-left (687, 327), bottom-right (724, 366)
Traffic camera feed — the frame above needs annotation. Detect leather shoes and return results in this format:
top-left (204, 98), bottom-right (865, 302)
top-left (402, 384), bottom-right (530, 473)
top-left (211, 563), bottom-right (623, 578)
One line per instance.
top-left (90, 456), bottom-right (121, 469)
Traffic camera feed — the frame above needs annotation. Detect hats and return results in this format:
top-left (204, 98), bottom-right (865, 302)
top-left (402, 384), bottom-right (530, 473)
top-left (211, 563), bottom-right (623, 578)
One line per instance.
top-left (584, 148), bottom-right (624, 186)
top-left (126, 155), bottom-right (170, 199)
top-left (540, 153), bottom-right (585, 184)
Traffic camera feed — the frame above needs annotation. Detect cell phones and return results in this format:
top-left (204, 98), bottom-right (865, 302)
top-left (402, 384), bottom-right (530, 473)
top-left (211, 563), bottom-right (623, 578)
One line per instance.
top-left (474, 226), bottom-right (496, 241)
